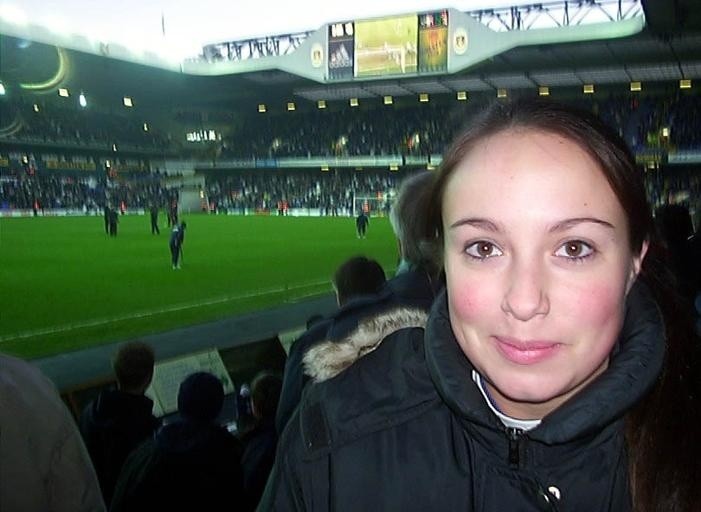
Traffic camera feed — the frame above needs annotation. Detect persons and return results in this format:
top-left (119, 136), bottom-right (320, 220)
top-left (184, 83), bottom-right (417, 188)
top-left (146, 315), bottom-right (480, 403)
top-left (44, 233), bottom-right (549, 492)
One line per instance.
top-left (99, 203), bottom-right (187, 270)
top-left (595, 95), bottom-right (700, 325)
top-left (121, 374), bottom-right (279, 512)
top-left (200, 107), bottom-right (463, 238)
top-left (80, 338), bottom-right (160, 497)
top-left (0, 85), bottom-right (184, 216)
top-left (254, 96), bottom-right (698, 512)
top-left (277, 170), bottom-right (449, 449)
top-left (1, 349), bottom-right (106, 512)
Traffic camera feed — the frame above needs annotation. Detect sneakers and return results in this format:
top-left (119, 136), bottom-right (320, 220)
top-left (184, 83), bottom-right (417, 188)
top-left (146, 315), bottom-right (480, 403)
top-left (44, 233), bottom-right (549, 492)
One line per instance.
top-left (172, 265), bottom-right (181, 270)
